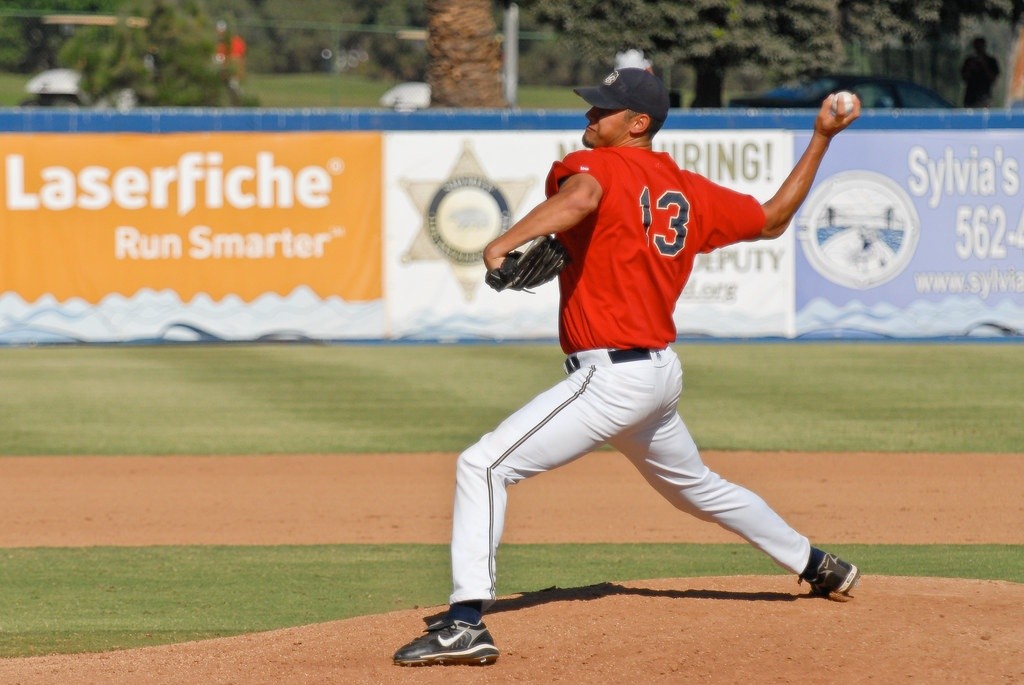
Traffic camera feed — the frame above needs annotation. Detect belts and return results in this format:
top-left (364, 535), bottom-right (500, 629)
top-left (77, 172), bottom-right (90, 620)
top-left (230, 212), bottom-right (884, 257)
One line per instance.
top-left (565, 347), bottom-right (652, 374)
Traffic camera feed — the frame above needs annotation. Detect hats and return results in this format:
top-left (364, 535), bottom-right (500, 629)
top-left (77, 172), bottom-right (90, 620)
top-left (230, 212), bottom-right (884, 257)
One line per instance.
top-left (573, 67), bottom-right (670, 121)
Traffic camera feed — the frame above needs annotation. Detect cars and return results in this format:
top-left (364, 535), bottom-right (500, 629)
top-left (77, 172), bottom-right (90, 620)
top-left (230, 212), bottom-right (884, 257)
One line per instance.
top-left (727, 73), bottom-right (960, 111)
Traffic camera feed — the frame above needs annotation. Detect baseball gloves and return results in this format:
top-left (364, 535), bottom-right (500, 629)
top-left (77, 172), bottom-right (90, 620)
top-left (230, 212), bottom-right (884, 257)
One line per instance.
top-left (481, 231), bottom-right (572, 294)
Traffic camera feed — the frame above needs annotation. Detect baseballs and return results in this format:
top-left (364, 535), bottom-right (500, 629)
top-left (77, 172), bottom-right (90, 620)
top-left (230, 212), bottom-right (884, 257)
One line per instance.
top-left (829, 89), bottom-right (858, 119)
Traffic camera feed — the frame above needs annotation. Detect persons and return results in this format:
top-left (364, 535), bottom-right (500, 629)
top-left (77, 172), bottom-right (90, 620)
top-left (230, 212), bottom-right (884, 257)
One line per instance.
top-left (215, 21), bottom-right (248, 103)
top-left (394, 66), bottom-right (863, 667)
top-left (958, 38), bottom-right (1000, 110)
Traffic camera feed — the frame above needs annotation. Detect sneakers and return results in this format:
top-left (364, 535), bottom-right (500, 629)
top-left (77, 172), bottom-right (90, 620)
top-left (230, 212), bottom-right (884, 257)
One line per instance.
top-left (393, 617), bottom-right (500, 665)
top-left (803, 551), bottom-right (861, 597)
